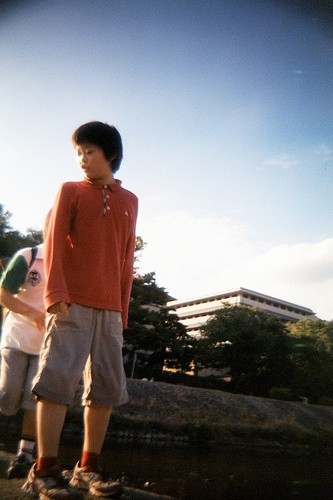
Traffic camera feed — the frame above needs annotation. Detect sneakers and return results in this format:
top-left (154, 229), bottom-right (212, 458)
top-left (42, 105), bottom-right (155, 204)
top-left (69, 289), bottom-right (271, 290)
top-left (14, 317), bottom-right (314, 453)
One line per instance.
top-left (7, 453), bottom-right (32, 479)
top-left (67, 460), bottom-right (121, 499)
top-left (20, 462), bottom-right (71, 500)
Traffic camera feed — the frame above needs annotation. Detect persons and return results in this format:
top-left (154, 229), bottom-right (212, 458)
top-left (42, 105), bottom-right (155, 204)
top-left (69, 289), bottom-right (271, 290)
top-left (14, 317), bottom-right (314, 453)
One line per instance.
top-left (1, 240), bottom-right (49, 478)
top-left (22, 120), bottom-right (139, 500)
